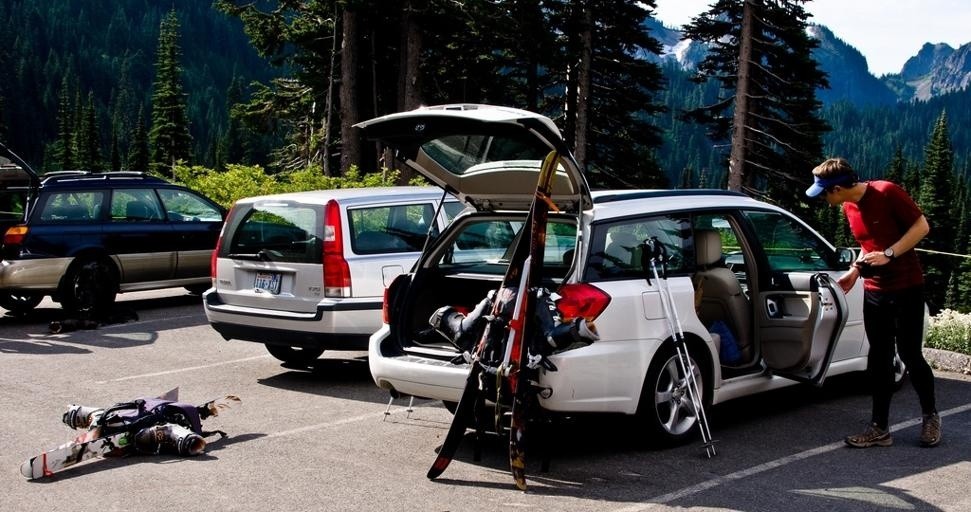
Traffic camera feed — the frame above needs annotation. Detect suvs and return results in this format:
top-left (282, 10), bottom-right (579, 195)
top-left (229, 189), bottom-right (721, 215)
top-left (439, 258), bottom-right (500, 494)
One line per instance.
top-left (347, 101), bottom-right (932, 449)
top-left (197, 177), bottom-right (523, 363)
top-left (0, 139), bottom-right (236, 331)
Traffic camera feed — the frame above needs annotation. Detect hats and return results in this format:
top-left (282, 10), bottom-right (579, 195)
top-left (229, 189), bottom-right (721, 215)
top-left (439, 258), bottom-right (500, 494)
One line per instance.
top-left (805, 174), bottom-right (856, 197)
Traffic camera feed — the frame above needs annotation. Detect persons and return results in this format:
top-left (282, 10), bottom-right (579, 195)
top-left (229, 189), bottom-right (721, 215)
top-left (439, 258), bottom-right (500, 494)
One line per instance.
top-left (804, 158), bottom-right (942, 449)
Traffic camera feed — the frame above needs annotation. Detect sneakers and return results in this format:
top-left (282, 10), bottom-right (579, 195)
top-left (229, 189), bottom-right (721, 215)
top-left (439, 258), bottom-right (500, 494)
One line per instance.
top-left (921, 413), bottom-right (942, 445)
top-left (845, 424), bottom-right (893, 447)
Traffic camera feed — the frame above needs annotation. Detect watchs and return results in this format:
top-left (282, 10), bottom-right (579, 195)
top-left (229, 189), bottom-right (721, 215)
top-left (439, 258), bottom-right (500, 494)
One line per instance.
top-left (884, 247), bottom-right (896, 262)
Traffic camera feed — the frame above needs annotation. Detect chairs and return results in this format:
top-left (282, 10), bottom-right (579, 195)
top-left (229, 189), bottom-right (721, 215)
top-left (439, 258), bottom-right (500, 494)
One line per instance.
top-left (688, 226), bottom-right (752, 363)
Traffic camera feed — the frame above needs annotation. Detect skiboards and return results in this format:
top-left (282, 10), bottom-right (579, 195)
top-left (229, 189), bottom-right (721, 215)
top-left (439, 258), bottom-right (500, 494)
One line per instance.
top-left (426, 151), bottom-right (562, 491)
top-left (20, 395), bottom-right (242, 479)
